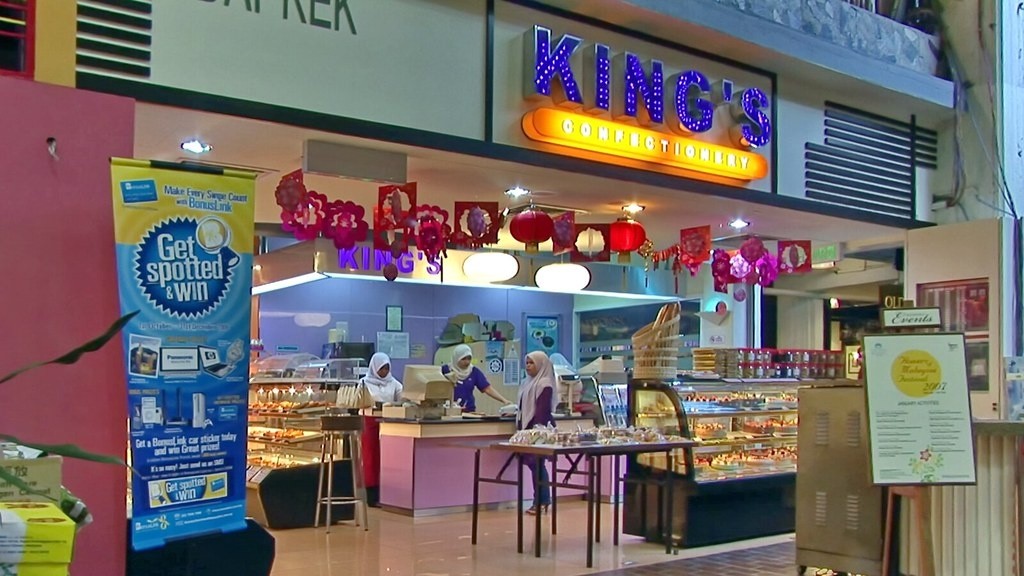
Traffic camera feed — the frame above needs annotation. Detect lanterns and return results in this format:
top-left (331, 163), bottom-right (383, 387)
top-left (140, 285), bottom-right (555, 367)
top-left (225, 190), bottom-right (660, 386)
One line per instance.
top-left (510, 207), bottom-right (555, 255)
top-left (610, 217), bottom-right (646, 263)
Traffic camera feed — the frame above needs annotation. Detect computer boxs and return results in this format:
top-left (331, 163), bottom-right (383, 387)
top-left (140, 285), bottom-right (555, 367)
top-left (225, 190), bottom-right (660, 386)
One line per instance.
top-left (193, 392), bottom-right (205, 428)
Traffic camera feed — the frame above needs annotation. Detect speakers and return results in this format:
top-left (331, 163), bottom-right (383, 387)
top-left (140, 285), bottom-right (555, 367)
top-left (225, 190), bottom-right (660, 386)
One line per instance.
top-left (141, 396), bottom-right (156, 423)
top-left (259, 459), bottom-right (354, 529)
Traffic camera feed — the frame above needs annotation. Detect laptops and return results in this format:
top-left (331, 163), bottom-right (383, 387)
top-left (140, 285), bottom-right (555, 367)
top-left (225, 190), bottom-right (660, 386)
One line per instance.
top-left (159, 346), bottom-right (200, 377)
top-left (197, 345), bottom-right (238, 378)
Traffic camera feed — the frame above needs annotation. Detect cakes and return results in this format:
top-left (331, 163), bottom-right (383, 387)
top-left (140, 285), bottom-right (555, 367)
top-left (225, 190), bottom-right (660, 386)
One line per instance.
top-left (682, 392), bottom-right (798, 469)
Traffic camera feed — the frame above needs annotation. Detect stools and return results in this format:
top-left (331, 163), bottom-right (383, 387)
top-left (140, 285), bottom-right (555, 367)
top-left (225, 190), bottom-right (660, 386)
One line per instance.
top-left (312, 413), bottom-right (372, 535)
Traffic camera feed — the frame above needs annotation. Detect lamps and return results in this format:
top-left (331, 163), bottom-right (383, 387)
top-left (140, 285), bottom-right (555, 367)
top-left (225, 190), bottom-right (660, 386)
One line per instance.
top-left (460, 242), bottom-right (521, 284)
top-left (533, 254), bottom-right (593, 293)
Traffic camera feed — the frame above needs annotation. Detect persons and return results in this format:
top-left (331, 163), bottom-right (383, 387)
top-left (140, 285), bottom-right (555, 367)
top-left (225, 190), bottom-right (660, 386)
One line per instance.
top-left (501, 351), bottom-right (557, 514)
top-left (356, 352), bottom-right (404, 406)
top-left (442, 343), bottom-right (513, 415)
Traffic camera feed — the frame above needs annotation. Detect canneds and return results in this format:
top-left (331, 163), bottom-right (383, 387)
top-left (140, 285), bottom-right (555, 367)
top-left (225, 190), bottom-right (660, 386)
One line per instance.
top-left (713, 348), bottom-right (846, 379)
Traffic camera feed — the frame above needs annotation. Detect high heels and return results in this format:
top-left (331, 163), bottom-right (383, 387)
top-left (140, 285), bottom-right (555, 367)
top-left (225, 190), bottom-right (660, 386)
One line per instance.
top-left (525, 502), bottom-right (551, 514)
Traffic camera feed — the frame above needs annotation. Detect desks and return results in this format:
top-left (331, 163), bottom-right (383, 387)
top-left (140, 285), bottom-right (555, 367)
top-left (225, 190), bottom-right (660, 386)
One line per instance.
top-left (437, 433), bottom-right (603, 554)
top-left (486, 438), bottom-right (702, 566)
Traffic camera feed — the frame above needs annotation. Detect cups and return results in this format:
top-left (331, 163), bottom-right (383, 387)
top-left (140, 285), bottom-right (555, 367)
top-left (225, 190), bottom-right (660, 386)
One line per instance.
top-left (737, 350), bottom-right (845, 378)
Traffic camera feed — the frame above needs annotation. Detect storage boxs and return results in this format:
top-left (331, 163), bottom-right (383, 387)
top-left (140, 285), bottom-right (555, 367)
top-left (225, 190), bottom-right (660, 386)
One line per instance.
top-left (0, 441), bottom-right (64, 509)
top-left (0, 562), bottom-right (69, 576)
top-left (0, 500), bottom-right (78, 564)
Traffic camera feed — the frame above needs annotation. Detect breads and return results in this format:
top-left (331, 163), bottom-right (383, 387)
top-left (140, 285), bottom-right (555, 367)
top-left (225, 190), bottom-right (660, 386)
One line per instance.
top-left (510, 427), bottom-right (683, 446)
top-left (249, 400), bottom-right (333, 468)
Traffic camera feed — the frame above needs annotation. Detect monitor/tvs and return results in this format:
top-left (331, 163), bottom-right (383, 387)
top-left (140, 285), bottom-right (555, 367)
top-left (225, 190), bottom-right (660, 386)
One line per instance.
top-left (400, 364), bottom-right (452, 405)
